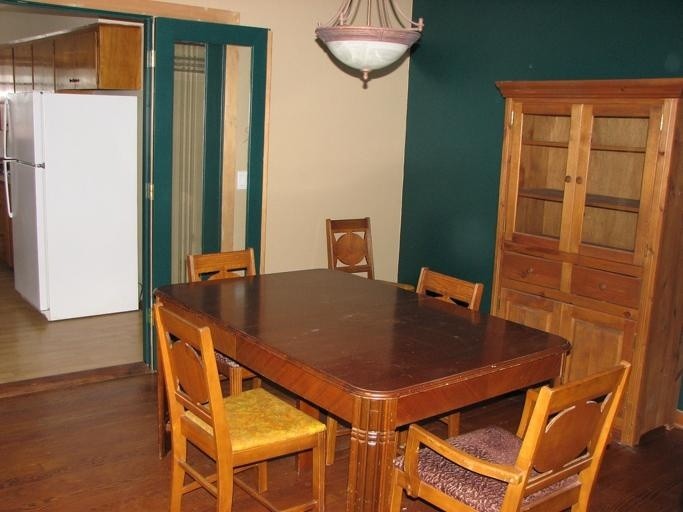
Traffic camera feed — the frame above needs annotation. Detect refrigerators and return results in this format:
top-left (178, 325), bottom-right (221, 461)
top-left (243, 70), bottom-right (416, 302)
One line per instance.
top-left (3, 91), bottom-right (139, 321)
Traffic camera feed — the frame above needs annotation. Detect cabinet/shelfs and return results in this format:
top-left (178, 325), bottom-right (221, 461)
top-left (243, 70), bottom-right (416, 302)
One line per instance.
top-left (489, 77), bottom-right (683, 450)
top-left (55, 23), bottom-right (139, 90)
top-left (0, 43), bottom-right (14, 92)
top-left (0, 35), bottom-right (55, 92)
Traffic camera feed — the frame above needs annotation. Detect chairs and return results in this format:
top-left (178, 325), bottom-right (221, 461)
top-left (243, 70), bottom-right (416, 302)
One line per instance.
top-left (389, 360), bottom-right (632, 511)
top-left (188, 247), bottom-right (274, 397)
top-left (326, 268), bottom-right (484, 466)
top-left (151, 303), bottom-right (329, 511)
top-left (326, 218), bottom-right (415, 291)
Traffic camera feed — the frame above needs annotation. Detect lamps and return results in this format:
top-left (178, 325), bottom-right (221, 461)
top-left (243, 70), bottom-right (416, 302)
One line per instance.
top-left (314, 0), bottom-right (424, 82)
